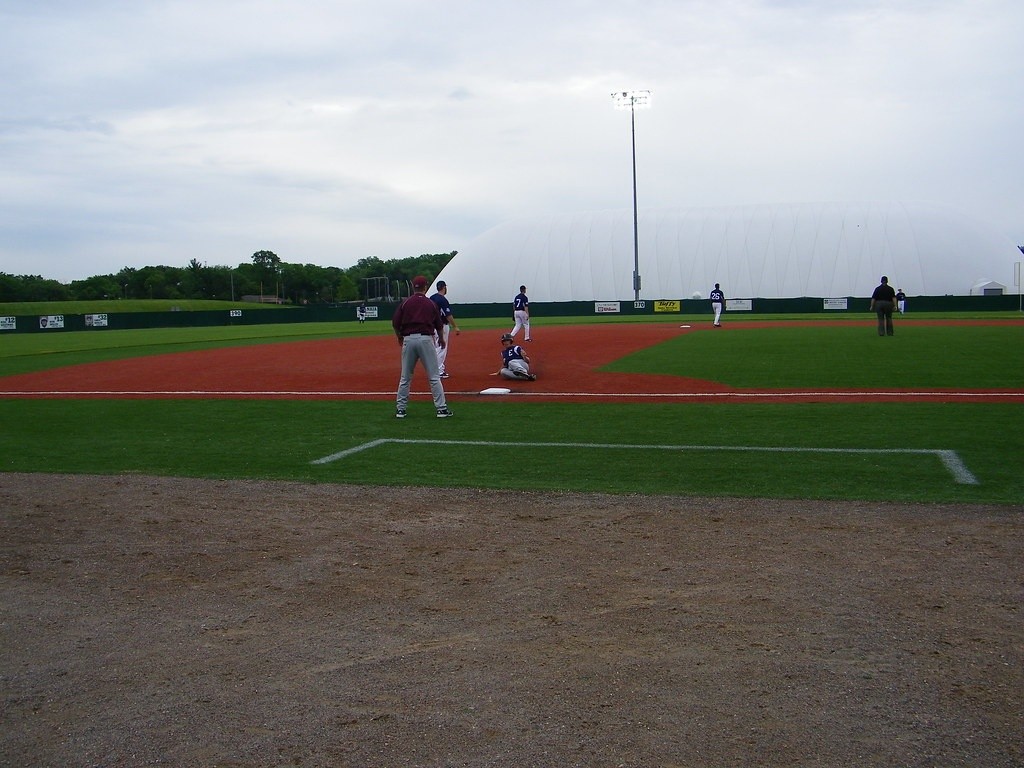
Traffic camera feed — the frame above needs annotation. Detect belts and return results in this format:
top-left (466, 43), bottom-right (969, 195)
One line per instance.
top-left (404, 332), bottom-right (430, 336)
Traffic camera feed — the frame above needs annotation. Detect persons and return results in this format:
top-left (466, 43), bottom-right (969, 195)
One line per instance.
top-left (710, 283), bottom-right (725, 327)
top-left (489, 333), bottom-right (536, 381)
top-left (511, 285), bottom-right (532, 341)
top-left (870, 276), bottom-right (898, 336)
top-left (359, 302), bottom-right (366, 323)
top-left (895, 289), bottom-right (906, 314)
top-left (392, 275), bottom-right (453, 418)
top-left (429, 281), bottom-right (461, 379)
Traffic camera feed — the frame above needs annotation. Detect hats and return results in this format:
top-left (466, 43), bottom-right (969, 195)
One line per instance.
top-left (881, 276), bottom-right (888, 283)
top-left (412, 276), bottom-right (427, 287)
top-left (436, 281), bottom-right (447, 289)
top-left (898, 289), bottom-right (901, 291)
top-left (520, 285), bottom-right (527, 291)
top-left (715, 284), bottom-right (719, 288)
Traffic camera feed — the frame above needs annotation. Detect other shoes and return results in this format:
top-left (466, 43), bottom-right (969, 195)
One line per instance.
top-left (528, 373), bottom-right (536, 380)
top-left (713, 324), bottom-right (721, 327)
top-left (524, 339), bottom-right (532, 342)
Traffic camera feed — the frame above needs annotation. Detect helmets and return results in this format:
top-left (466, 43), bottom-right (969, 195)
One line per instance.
top-left (501, 333), bottom-right (514, 342)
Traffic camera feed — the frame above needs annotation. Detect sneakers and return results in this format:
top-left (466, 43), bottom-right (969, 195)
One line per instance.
top-left (437, 409), bottom-right (453, 417)
top-left (440, 373), bottom-right (449, 378)
top-left (396, 409), bottom-right (407, 418)
